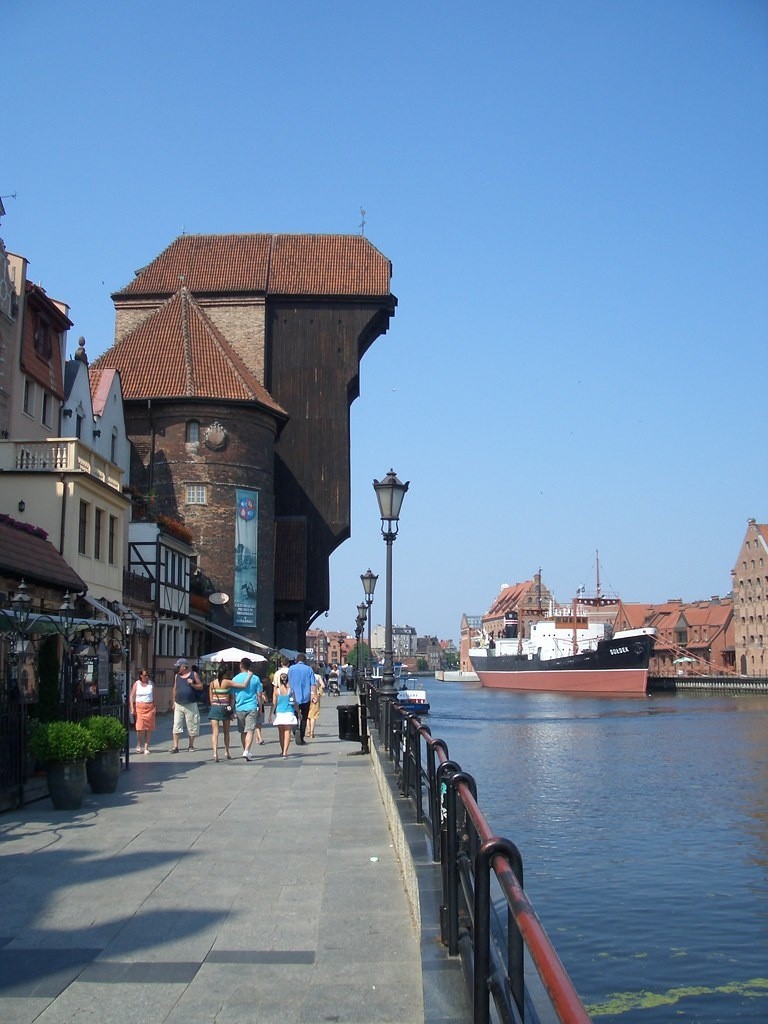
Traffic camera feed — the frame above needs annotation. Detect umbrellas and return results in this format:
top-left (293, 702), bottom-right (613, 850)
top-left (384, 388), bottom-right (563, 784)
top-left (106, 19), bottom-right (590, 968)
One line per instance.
top-left (200, 647), bottom-right (269, 676)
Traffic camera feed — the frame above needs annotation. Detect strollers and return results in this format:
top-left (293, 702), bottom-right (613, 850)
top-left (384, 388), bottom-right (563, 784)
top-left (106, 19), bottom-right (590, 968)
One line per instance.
top-left (328, 673), bottom-right (340, 697)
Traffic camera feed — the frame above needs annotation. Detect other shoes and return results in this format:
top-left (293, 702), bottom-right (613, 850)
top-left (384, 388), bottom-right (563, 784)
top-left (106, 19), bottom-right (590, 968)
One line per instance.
top-left (168, 747), bottom-right (179, 753)
top-left (188, 746), bottom-right (194, 752)
top-left (295, 729), bottom-right (305, 744)
top-left (144, 750), bottom-right (150, 755)
top-left (280, 752), bottom-right (288, 757)
top-left (225, 753), bottom-right (231, 759)
top-left (214, 757), bottom-right (218, 762)
top-left (257, 739), bottom-right (264, 744)
top-left (243, 751), bottom-right (252, 761)
top-left (136, 746), bottom-right (141, 753)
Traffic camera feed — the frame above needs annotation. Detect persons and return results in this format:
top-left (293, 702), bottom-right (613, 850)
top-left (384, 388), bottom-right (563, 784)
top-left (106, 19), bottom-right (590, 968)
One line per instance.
top-left (168, 658), bottom-right (202, 753)
top-left (207, 653), bottom-right (325, 763)
top-left (318, 662), bottom-right (352, 692)
top-left (402, 684), bottom-right (407, 690)
top-left (130, 670), bottom-right (157, 754)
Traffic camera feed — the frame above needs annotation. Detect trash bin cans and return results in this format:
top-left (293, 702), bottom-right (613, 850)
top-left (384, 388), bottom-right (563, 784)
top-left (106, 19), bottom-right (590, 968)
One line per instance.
top-left (346, 677), bottom-right (354, 691)
top-left (336, 704), bottom-right (360, 741)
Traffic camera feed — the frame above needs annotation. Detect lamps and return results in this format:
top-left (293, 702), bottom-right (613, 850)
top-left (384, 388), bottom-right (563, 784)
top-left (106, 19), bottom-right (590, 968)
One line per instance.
top-left (93, 429), bottom-right (101, 438)
top-left (63, 409), bottom-right (72, 418)
top-left (18, 500), bottom-right (25, 512)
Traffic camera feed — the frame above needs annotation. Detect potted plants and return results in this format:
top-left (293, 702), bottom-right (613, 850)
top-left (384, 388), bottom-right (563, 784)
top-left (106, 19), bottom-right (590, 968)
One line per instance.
top-left (27, 720), bottom-right (103, 811)
top-left (79, 714), bottom-right (129, 794)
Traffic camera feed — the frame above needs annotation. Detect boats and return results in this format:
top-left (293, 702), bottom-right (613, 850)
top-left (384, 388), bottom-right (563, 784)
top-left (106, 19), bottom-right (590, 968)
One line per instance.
top-left (371, 661), bottom-right (431, 715)
top-left (468, 549), bottom-right (658, 693)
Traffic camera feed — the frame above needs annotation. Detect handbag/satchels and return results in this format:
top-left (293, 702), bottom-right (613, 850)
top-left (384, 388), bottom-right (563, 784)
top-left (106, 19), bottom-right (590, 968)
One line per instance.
top-left (221, 705), bottom-right (234, 716)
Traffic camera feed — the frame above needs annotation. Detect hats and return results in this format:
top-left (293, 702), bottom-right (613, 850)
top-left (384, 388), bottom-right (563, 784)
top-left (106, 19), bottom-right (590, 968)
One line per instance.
top-left (174, 658), bottom-right (188, 666)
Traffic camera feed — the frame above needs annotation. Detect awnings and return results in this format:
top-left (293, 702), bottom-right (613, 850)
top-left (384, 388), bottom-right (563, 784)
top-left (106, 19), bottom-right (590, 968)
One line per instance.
top-left (78, 594), bottom-right (146, 629)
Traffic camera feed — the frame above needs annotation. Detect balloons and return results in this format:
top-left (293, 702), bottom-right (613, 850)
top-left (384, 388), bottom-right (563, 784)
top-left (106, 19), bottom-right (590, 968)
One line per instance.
top-left (238, 497), bottom-right (255, 520)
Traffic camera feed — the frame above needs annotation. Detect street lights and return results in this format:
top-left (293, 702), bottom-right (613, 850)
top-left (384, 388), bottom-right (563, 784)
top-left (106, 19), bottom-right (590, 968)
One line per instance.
top-left (59, 590), bottom-right (77, 723)
top-left (120, 610), bottom-right (139, 769)
top-left (372, 468), bottom-right (411, 699)
top-left (354, 601), bottom-right (368, 675)
top-left (337, 635), bottom-right (345, 666)
top-left (11, 579), bottom-right (34, 809)
